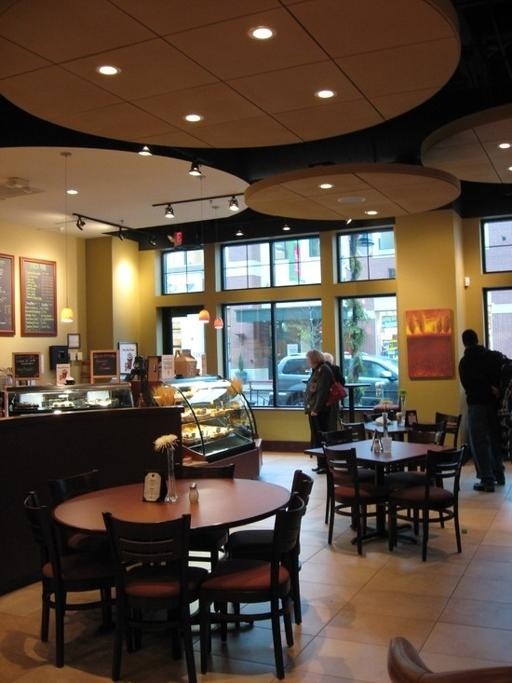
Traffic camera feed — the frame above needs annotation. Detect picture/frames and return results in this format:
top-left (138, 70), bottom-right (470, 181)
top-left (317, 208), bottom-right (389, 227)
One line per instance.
top-left (88, 350), bottom-right (118, 385)
top-left (161, 354), bottom-right (175, 381)
top-left (147, 355), bottom-right (162, 383)
top-left (118, 340), bottom-right (139, 375)
top-left (67, 333), bottom-right (81, 349)
top-left (55, 363), bottom-right (70, 386)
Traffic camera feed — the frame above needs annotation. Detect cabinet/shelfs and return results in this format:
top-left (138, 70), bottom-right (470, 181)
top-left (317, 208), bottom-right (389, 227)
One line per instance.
top-left (157, 374), bottom-right (260, 481)
top-left (0, 402), bottom-right (185, 598)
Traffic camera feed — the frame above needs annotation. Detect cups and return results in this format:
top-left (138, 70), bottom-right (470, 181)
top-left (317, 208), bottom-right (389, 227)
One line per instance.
top-left (382, 437), bottom-right (391, 454)
top-left (396, 412), bottom-right (405, 430)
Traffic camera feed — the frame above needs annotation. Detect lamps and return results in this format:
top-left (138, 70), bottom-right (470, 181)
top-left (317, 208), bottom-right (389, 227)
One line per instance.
top-left (76, 217), bottom-right (86, 231)
top-left (186, 157), bottom-right (202, 176)
top-left (137, 144), bottom-right (152, 157)
top-left (234, 227), bottom-right (245, 238)
top-left (147, 235), bottom-right (160, 247)
top-left (213, 319), bottom-right (225, 330)
top-left (229, 194), bottom-right (241, 211)
top-left (163, 202), bottom-right (176, 221)
top-left (117, 228), bottom-right (127, 241)
top-left (281, 220), bottom-right (293, 232)
top-left (199, 308), bottom-right (211, 323)
top-left (60, 307), bottom-right (75, 325)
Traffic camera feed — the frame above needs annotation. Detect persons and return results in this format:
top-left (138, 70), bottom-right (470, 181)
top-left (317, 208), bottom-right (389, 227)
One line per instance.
top-left (459, 329), bottom-right (505, 494)
top-left (305, 350), bottom-right (337, 474)
top-left (321, 353), bottom-right (344, 457)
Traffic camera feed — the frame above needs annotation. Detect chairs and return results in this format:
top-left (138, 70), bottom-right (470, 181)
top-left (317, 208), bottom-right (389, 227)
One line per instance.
top-left (25, 491), bottom-right (124, 668)
top-left (102, 509), bottom-right (210, 683)
top-left (363, 412), bottom-right (394, 424)
top-left (391, 422), bottom-right (448, 536)
top-left (338, 420), bottom-right (366, 441)
top-left (170, 462), bottom-right (234, 611)
top-left (225, 468), bottom-right (313, 627)
top-left (63, 466), bottom-right (123, 552)
top-left (387, 446), bottom-right (465, 562)
top-left (319, 428), bottom-right (380, 528)
top-left (323, 447), bottom-right (394, 555)
top-left (433, 411), bottom-right (463, 448)
top-left (380, 631), bottom-right (512, 683)
top-left (193, 495), bottom-right (305, 680)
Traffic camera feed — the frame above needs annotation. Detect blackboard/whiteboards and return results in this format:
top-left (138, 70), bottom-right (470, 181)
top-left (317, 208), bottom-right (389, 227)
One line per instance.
top-left (89, 349), bottom-right (120, 378)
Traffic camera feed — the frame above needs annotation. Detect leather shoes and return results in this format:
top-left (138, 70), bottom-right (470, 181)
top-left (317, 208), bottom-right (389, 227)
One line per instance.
top-left (317, 468), bottom-right (327, 474)
top-left (312, 467), bottom-right (319, 471)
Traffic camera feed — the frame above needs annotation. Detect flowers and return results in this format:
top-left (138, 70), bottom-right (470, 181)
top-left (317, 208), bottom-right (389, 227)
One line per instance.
top-left (399, 388), bottom-right (409, 401)
top-left (381, 400), bottom-right (392, 430)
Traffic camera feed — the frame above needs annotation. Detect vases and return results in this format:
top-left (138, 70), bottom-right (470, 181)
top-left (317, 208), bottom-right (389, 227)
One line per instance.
top-left (400, 396), bottom-right (408, 426)
top-left (382, 411), bottom-right (390, 442)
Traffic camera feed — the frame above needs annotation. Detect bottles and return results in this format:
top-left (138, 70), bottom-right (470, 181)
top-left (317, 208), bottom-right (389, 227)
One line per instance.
top-left (189, 482), bottom-right (198, 505)
top-left (374, 439), bottom-right (380, 454)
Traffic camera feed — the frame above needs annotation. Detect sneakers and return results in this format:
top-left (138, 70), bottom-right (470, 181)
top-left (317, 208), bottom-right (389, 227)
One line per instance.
top-left (474, 478), bottom-right (495, 492)
top-left (495, 474), bottom-right (505, 485)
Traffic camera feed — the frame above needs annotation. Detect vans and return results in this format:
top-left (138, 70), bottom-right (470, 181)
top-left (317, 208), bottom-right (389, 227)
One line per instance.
top-left (277, 352), bottom-right (399, 406)
